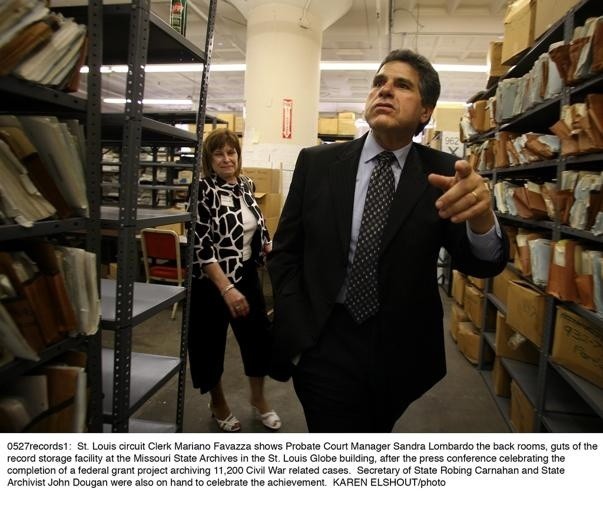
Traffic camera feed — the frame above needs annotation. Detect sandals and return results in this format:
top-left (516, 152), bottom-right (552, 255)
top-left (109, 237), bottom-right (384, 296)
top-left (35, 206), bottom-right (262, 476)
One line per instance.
top-left (208, 401), bottom-right (241, 431)
top-left (250, 402), bottom-right (281, 429)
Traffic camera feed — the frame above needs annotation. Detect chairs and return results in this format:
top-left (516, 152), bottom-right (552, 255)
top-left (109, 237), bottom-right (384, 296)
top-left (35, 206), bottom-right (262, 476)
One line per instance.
top-left (140, 226), bottom-right (186, 322)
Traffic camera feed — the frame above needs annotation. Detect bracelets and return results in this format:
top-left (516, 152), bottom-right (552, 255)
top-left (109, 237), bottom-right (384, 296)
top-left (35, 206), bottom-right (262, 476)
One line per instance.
top-left (220, 283), bottom-right (236, 295)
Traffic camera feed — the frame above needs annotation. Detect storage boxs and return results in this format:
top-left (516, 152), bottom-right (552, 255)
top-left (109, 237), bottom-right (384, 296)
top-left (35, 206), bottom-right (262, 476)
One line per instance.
top-left (532, 0), bottom-right (582, 42)
top-left (235, 114), bottom-right (245, 133)
top-left (155, 222), bottom-right (184, 235)
top-left (492, 357), bottom-right (508, 399)
top-left (505, 278), bottom-right (544, 350)
top-left (495, 310), bottom-right (529, 363)
top-left (509, 379), bottom-right (537, 433)
top-left (450, 305), bottom-right (467, 344)
top-left (466, 275), bottom-right (489, 292)
top-left (435, 105), bottom-right (468, 133)
top-left (550, 306), bottom-right (603, 386)
top-left (264, 217), bottom-right (279, 240)
top-left (251, 193), bottom-right (282, 220)
top-left (457, 323), bottom-right (482, 365)
top-left (175, 170), bottom-right (193, 186)
top-left (337, 112), bottom-right (358, 137)
top-left (441, 130), bottom-right (464, 159)
top-left (452, 270), bottom-right (465, 306)
top-left (318, 117), bottom-right (339, 138)
top-left (215, 113), bottom-right (234, 133)
top-left (486, 40), bottom-right (510, 89)
top-left (492, 270), bottom-right (519, 306)
top-left (243, 168), bottom-right (280, 195)
top-left (189, 123), bottom-right (213, 141)
top-left (502, 1), bottom-right (534, 64)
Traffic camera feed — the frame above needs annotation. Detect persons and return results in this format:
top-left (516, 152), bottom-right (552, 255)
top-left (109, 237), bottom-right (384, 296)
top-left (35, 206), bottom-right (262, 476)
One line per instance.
top-left (184, 127), bottom-right (283, 431)
top-left (268, 50), bottom-right (510, 433)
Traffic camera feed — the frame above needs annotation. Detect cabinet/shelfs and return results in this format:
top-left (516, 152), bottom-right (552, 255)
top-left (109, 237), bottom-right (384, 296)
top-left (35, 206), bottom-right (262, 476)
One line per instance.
top-left (460, 1), bottom-right (603, 434)
top-left (97, 113), bottom-right (227, 206)
top-left (0, 0), bottom-right (219, 434)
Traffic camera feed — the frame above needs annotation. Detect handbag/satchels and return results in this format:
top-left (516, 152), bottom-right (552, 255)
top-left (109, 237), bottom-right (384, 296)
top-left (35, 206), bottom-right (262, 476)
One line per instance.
top-left (255, 260), bottom-right (276, 337)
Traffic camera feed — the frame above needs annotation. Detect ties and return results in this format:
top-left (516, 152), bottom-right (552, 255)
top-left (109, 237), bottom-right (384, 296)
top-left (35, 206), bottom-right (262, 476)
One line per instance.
top-left (343, 151), bottom-right (398, 323)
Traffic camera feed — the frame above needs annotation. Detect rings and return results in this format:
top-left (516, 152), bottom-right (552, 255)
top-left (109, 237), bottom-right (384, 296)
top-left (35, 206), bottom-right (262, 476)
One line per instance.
top-left (472, 191), bottom-right (480, 204)
